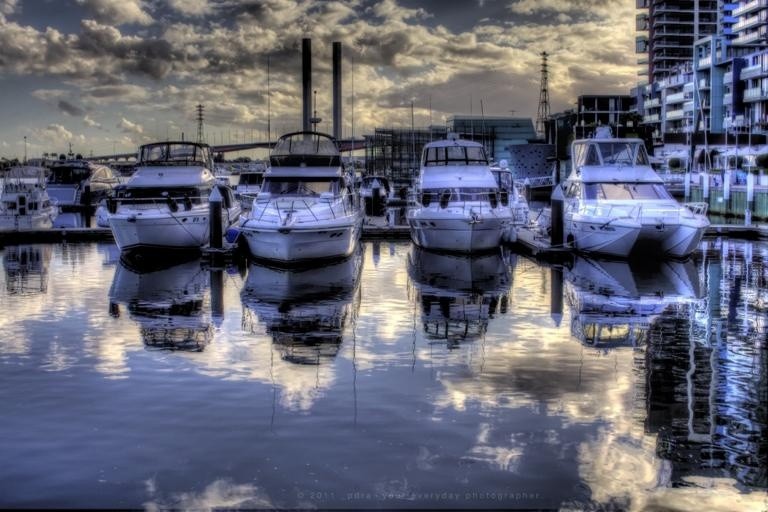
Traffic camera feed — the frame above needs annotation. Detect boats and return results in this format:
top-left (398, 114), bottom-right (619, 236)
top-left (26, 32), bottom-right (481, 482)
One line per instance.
top-left (236, 131), bottom-right (366, 262)
top-left (404, 140), bottom-right (514, 251)
top-left (538, 124), bottom-right (710, 260)
top-left (107, 249), bottom-right (217, 353)
top-left (562, 253), bottom-right (708, 357)
top-left (404, 240), bottom-right (515, 349)
top-left (239, 255), bottom-right (365, 368)
top-left (1, 239), bottom-right (56, 300)
top-left (109, 142), bottom-right (242, 255)
